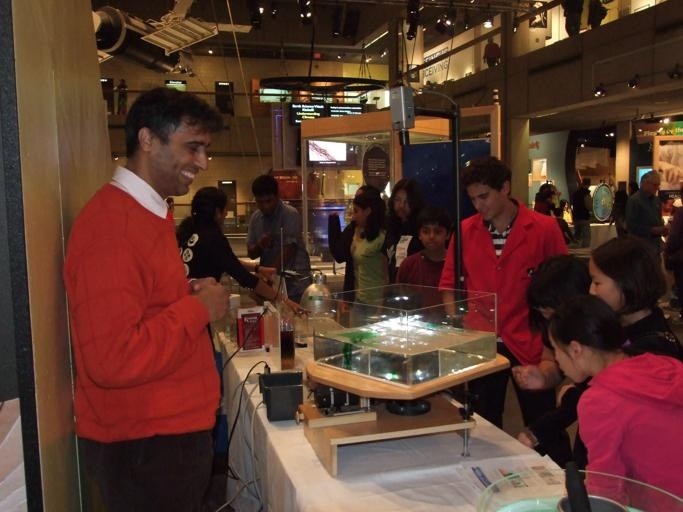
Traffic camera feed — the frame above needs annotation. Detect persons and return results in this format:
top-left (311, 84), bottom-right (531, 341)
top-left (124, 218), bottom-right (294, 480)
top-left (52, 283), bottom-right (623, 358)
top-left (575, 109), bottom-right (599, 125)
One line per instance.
top-left (175, 187), bottom-right (311, 319)
top-left (588, 0), bottom-right (606, 29)
top-left (483, 37), bottom-right (501, 68)
top-left (113, 78), bottom-right (128, 115)
top-left (246, 174), bottom-right (313, 304)
top-left (560, 0), bottom-right (584, 37)
top-left (64, 87), bottom-right (224, 512)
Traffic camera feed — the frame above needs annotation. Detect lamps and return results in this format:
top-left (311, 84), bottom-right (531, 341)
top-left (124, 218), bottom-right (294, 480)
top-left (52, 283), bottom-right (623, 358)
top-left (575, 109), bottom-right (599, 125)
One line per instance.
top-left (586, 62), bottom-right (682, 99)
top-left (239, 0), bottom-right (498, 48)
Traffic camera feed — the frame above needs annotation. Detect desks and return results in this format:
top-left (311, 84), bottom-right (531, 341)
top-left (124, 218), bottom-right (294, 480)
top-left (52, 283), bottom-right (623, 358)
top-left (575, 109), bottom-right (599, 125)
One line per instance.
top-left (219, 308), bottom-right (566, 511)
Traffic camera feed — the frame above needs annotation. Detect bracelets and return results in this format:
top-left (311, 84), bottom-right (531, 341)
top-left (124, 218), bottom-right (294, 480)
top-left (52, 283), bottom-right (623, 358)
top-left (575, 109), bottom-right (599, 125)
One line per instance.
top-left (255, 264), bottom-right (260, 273)
